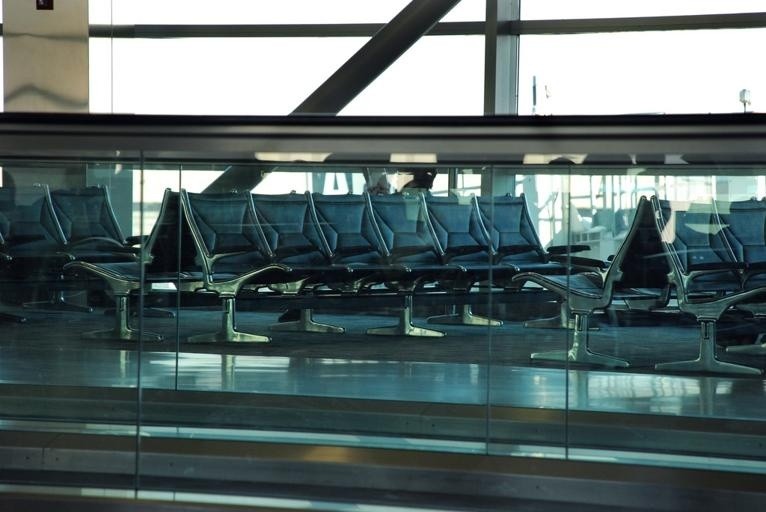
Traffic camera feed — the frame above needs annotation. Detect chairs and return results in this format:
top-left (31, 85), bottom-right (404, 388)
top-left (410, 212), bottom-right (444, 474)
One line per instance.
top-left (0, 184), bottom-right (766, 379)
